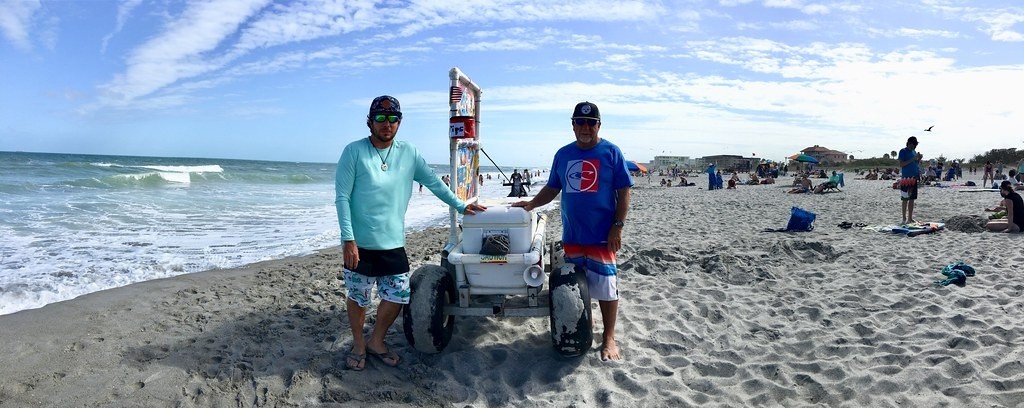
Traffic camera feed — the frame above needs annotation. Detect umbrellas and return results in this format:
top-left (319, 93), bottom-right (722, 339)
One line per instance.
top-left (626, 161), bottom-right (647, 172)
top-left (790, 154), bottom-right (819, 163)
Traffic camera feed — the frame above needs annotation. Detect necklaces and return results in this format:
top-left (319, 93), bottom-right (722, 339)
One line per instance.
top-left (369, 136), bottom-right (394, 171)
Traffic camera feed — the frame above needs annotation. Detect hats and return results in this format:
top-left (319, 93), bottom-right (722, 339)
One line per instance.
top-left (999, 181), bottom-right (1012, 188)
top-left (572, 102), bottom-right (601, 121)
top-left (370, 95), bottom-right (402, 115)
top-left (907, 135), bottom-right (919, 143)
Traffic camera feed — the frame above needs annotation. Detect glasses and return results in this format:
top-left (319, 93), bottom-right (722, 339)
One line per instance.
top-left (373, 114), bottom-right (400, 124)
top-left (574, 117), bottom-right (599, 127)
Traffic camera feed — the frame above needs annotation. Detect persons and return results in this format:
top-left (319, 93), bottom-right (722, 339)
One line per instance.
top-left (898, 136), bottom-right (923, 225)
top-left (982, 158), bottom-right (1024, 189)
top-left (756, 164), bottom-right (788, 184)
top-left (510, 169), bottom-right (540, 191)
top-left (441, 175), bottom-right (450, 187)
top-left (335, 95), bottom-right (487, 372)
top-left (479, 173), bottom-right (500, 186)
top-left (707, 163), bottom-right (739, 190)
top-left (794, 163), bottom-right (839, 193)
top-left (419, 183), bottom-right (423, 193)
top-left (918, 159), bottom-right (959, 184)
top-left (969, 166), bottom-right (977, 176)
top-left (865, 167), bottom-right (899, 181)
top-left (987, 181), bottom-right (1024, 233)
top-left (659, 164), bottom-right (687, 186)
top-left (512, 101), bottom-right (634, 361)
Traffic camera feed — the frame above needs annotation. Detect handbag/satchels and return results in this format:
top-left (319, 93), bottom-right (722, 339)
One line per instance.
top-left (786, 207), bottom-right (816, 230)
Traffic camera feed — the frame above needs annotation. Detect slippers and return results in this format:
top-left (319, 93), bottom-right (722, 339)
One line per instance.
top-left (348, 347), bottom-right (364, 370)
top-left (365, 341), bottom-right (401, 366)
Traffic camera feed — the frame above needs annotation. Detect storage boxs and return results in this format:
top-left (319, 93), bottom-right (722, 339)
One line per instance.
top-left (462, 198), bottom-right (538, 254)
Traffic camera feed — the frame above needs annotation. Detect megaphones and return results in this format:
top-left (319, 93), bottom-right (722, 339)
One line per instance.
top-left (522, 264), bottom-right (546, 287)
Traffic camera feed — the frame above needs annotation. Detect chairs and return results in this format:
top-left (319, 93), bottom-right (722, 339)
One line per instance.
top-left (828, 173), bottom-right (843, 192)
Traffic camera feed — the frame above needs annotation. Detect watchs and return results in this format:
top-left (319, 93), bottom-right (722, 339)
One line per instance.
top-left (613, 220), bottom-right (624, 227)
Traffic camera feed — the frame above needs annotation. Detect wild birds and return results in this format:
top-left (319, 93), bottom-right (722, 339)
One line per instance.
top-left (924, 125), bottom-right (935, 132)
